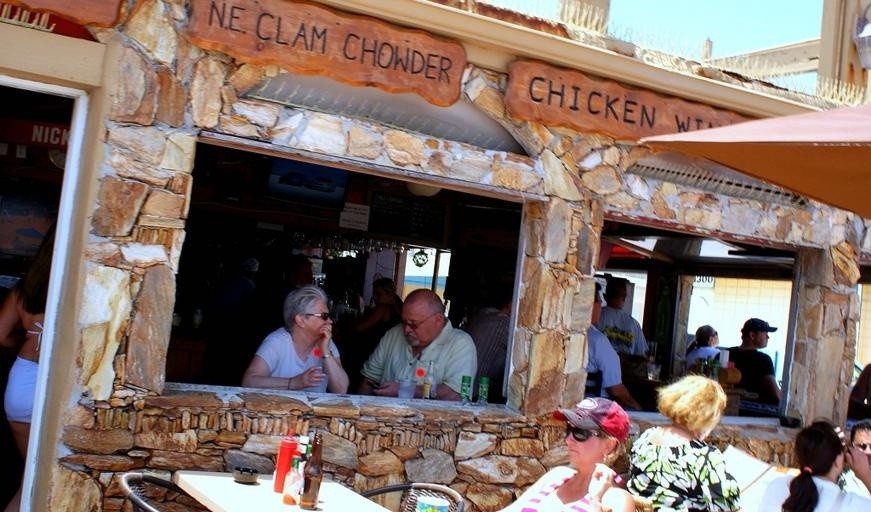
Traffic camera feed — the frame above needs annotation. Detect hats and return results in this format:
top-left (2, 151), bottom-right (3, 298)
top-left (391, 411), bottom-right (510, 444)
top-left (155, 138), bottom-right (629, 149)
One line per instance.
top-left (742, 318), bottom-right (778, 333)
top-left (552, 395), bottom-right (630, 443)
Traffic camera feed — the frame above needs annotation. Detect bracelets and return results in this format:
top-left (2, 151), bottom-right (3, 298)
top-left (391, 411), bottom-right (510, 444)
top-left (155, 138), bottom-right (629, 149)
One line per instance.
top-left (319, 351), bottom-right (332, 360)
top-left (286, 376), bottom-right (293, 390)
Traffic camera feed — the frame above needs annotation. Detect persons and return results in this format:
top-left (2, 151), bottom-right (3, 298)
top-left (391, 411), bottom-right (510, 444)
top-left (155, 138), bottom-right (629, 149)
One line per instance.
top-left (351, 277), bottom-right (404, 366)
top-left (493, 397), bottom-right (636, 512)
top-left (724, 316), bottom-right (781, 407)
top-left (357, 289), bottom-right (478, 403)
top-left (240, 284), bottom-right (352, 392)
top-left (755, 421), bottom-right (871, 512)
top-left (683, 324), bottom-right (721, 364)
top-left (465, 291), bottom-right (512, 401)
top-left (582, 282), bottom-right (642, 411)
top-left (849, 420), bottom-right (869, 456)
top-left (626, 374), bottom-right (743, 512)
top-left (593, 278), bottom-right (650, 358)
top-left (0, 219), bottom-right (58, 511)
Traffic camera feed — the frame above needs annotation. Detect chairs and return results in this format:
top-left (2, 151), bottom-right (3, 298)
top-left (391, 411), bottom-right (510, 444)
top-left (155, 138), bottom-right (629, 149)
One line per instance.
top-left (358, 483), bottom-right (466, 512)
top-left (119, 471), bottom-right (195, 511)
top-left (583, 370), bottom-right (603, 397)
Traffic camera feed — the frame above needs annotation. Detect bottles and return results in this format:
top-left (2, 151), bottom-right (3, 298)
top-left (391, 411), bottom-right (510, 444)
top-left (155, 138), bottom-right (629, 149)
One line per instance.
top-left (298, 436), bottom-right (310, 494)
top-left (460, 376), bottom-right (471, 400)
top-left (698, 358), bottom-right (704, 373)
top-left (706, 356), bottom-right (711, 378)
top-left (709, 364), bottom-right (718, 381)
top-left (422, 360), bottom-right (437, 399)
top-left (282, 450), bottom-right (301, 505)
top-left (299, 432), bottom-right (323, 511)
top-left (479, 376), bottom-right (489, 400)
top-left (274, 429), bottom-right (297, 493)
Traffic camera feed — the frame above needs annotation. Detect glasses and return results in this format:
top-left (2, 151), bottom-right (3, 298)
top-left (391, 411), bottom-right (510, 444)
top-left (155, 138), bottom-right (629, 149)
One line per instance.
top-left (849, 440), bottom-right (871, 456)
top-left (403, 311), bottom-right (440, 330)
top-left (564, 424), bottom-right (600, 441)
top-left (712, 331), bottom-right (718, 337)
top-left (304, 311), bottom-right (331, 321)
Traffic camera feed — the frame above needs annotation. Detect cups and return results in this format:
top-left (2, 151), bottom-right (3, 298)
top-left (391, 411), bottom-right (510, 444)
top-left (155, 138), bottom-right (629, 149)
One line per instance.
top-left (398, 379), bottom-right (417, 399)
top-left (313, 375), bottom-right (330, 392)
